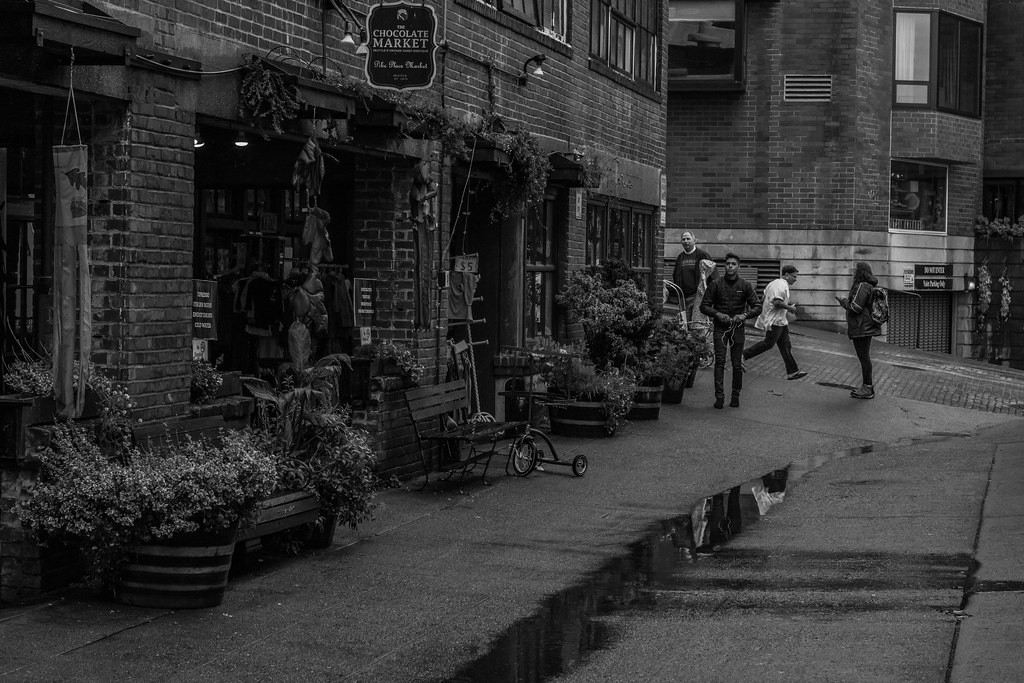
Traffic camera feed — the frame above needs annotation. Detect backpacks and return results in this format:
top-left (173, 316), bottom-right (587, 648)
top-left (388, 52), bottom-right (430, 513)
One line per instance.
top-left (866, 287), bottom-right (890, 330)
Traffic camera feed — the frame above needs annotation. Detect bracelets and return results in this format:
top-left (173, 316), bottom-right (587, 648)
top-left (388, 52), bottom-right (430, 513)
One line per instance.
top-left (743, 312), bottom-right (748, 319)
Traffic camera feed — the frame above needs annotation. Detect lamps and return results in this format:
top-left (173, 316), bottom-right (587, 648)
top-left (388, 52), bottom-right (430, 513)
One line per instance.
top-left (519, 52), bottom-right (545, 81)
top-left (235, 131), bottom-right (248, 146)
top-left (320, 0), bottom-right (369, 74)
top-left (194, 132), bottom-right (205, 148)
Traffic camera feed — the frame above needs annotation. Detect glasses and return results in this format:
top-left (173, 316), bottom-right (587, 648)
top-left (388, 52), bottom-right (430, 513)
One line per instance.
top-left (788, 273), bottom-right (797, 279)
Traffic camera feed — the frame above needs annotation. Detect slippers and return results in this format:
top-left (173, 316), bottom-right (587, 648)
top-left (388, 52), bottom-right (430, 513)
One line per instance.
top-left (787, 370), bottom-right (807, 380)
top-left (741, 361), bottom-right (747, 373)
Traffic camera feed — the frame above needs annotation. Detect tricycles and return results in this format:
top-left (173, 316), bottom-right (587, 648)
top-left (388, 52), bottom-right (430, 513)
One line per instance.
top-left (501, 344), bottom-right (591, 479)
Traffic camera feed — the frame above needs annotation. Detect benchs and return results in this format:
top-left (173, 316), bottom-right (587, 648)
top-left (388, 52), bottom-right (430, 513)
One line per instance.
top-left (404, 380), bottom-right (519, 494)
top-left (131, 417), bottom-right (341, 591)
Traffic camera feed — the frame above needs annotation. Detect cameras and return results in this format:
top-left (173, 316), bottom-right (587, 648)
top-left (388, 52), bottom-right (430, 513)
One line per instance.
top-left (730, 318), bottom-right (734, 322)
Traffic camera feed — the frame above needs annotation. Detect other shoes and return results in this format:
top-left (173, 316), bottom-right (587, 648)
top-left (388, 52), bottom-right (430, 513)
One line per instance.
top-left (714, 399), bottom-right (725, 409)
top-left (730, 397), bottom-right (740, 407)
top-left (851, 383), bottom-right (876, 399)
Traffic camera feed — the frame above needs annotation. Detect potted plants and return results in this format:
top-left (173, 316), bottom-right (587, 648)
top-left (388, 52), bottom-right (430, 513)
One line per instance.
top-left (350, 341), bottom-right (425, 383)
top-left (542, 259), bottom-right (707, 437)
top-left (0, 351), bottom-right (280, 610)
top-left (242, 46), bottom-right (616, 224)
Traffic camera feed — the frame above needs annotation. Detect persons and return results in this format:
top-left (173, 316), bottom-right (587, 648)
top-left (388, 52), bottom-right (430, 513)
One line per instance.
top-left (673, 231), bottom-right (720, 332)
top-left (838, 262), bottom-right (881, 399)
top-left (741, 265), bottom-right (808, 380)
top-left (700, 253), bottom-right (762, 409)
